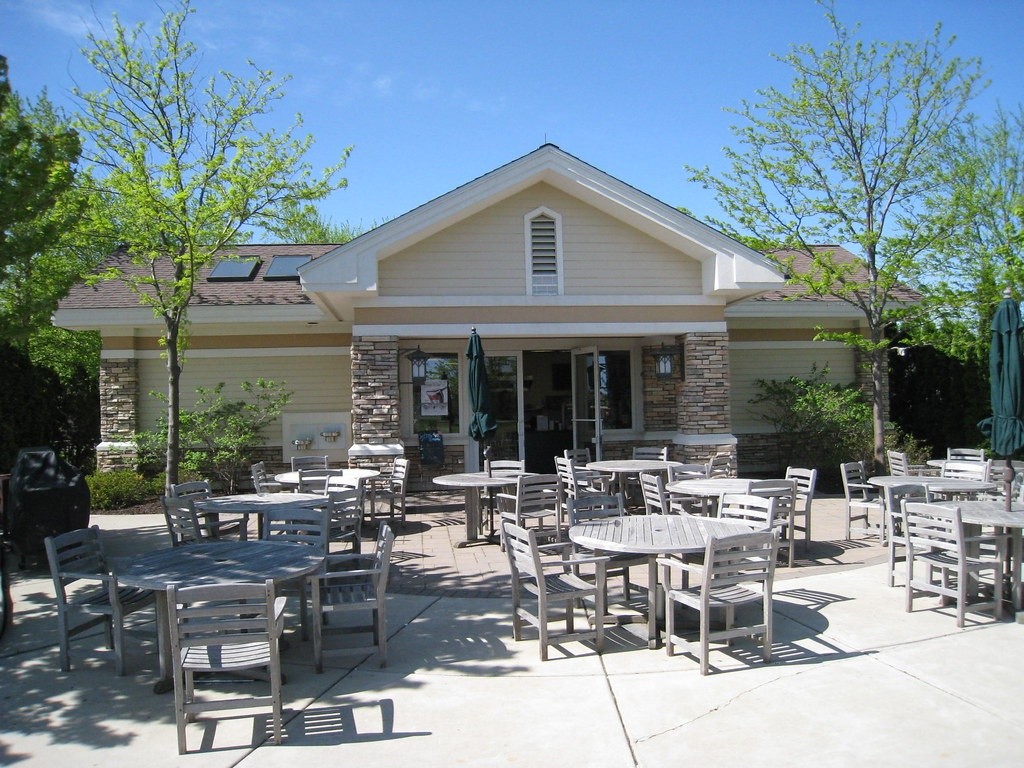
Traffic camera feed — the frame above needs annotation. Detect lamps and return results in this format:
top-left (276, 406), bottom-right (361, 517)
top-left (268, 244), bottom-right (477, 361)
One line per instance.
top-left (645, 342), bottom-right (685, 381)
top-left (397, 344), bottom-right (430, 386)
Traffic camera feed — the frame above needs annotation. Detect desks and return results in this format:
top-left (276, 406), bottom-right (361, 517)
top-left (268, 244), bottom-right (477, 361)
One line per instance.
top-left (926, 460), bottom-right (1024, 474)
top-left (867, 475), bottom-right (998, 492)
top-left (665, 478), bottom-right (802, 517)
top-left (194, 492), bottom-right (329, 541)
top-left (931, 501), bottom-right (1024, 620)
top-left (432, 471), bottom-right (541, 548)
top-left (115, 541), bottom-right (326, 694)
top-left (585, 460), bottom-right (682, 510)
top-left (274, 468), bottom-right (381, 531)
top-left (568, 514), bottom-right (753, 650)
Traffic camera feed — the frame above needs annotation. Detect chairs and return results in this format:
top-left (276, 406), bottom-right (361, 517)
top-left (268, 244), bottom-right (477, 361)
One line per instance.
top-left (42, 444), bottom-right (1024, 754)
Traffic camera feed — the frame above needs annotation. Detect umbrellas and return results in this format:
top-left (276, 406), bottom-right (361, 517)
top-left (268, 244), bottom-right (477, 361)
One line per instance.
top-left (977, 287), bottom-right (1024, 604)
top-left (454, 325), bottom-right (501, 549)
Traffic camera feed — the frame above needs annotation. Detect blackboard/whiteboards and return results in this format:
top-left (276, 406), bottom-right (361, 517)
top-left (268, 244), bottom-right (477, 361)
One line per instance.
top-left (418, 431), bottom-right (443, 466)
